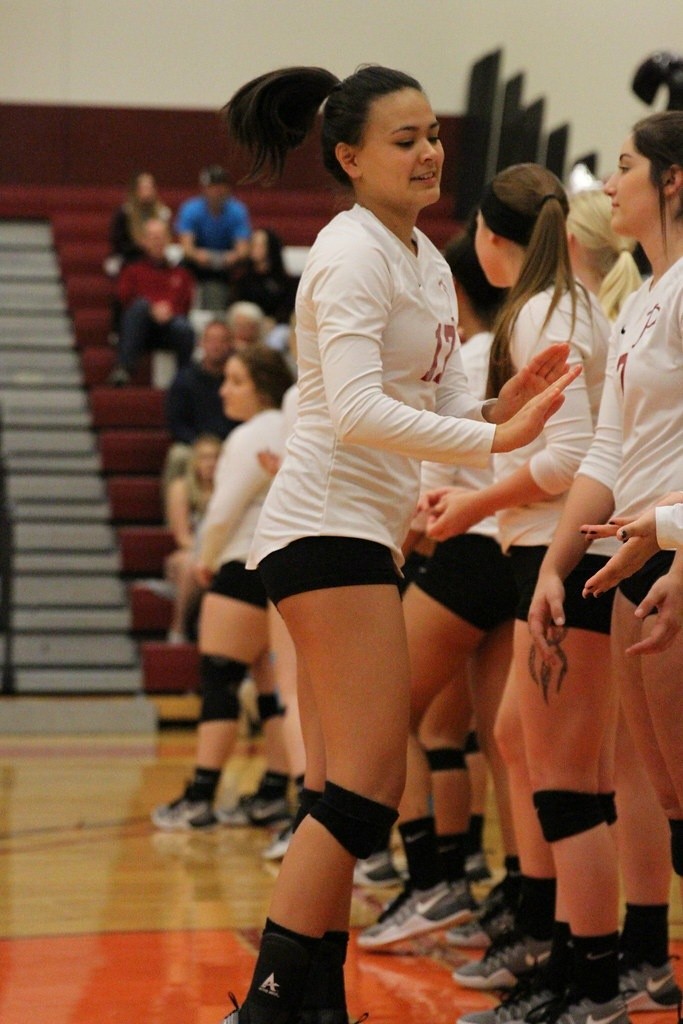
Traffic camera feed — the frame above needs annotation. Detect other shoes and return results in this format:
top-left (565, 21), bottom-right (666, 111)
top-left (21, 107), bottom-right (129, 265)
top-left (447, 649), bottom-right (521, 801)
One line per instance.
top-left (103, 365), bottom-right (131, 387)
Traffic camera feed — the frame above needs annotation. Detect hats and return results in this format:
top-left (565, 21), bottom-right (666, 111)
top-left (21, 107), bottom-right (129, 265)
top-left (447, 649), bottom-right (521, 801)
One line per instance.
top-left (200, 167), bottom-right (232, 186)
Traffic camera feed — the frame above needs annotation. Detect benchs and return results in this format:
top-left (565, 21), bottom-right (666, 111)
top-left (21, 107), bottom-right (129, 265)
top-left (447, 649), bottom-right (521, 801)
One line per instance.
top-left (48, 185), bottom-right (467, 692)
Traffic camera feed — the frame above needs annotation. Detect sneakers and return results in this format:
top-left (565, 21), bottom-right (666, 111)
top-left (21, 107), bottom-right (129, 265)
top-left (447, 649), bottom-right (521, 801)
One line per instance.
top-left (213, 793), bottom-right (295, 830)
top-left (607, 951), bottom-right (682, 1012)
top-left (261, 811), bottom-right (558, 989)
top-left (148, 799), bottom-right (222, 834)
top-left (448, 959), bottom-right (630, 1024)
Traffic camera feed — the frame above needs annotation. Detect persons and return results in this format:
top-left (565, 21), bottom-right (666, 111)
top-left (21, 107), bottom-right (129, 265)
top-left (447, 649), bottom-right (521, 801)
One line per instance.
top-left (150, 345), bottom-right (307, 859)
top-left (356, 109), bottom-right (683, 1024)
top-left (106, 171), bottom-right (298, 644)
top-left (218, 65), bottom-right (581, 1024)
